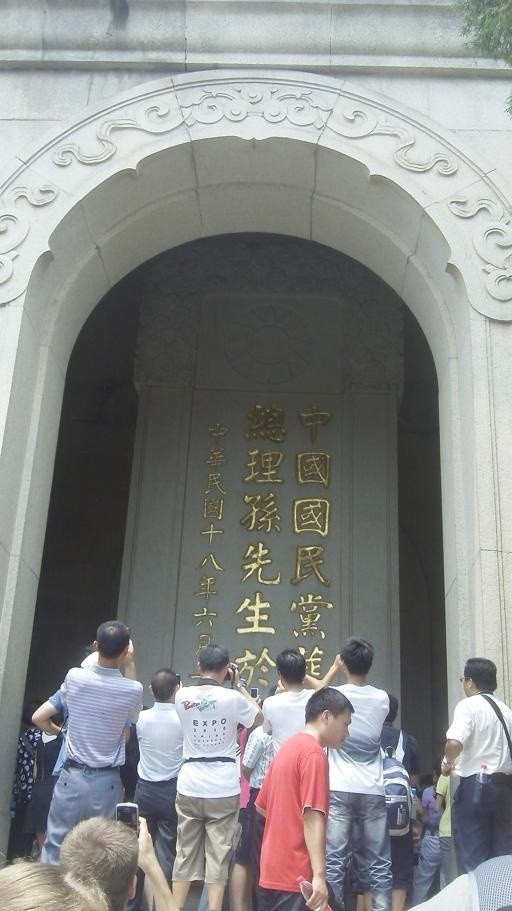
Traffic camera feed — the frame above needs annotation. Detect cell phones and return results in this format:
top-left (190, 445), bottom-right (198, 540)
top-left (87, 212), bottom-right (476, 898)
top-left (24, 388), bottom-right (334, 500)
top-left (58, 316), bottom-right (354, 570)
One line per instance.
top-left (175, 674), bottom-right (180, 685)
top-left (116, 802), bottom-right (140, 837)
top-left (250, 688), bottom-right (258, 698)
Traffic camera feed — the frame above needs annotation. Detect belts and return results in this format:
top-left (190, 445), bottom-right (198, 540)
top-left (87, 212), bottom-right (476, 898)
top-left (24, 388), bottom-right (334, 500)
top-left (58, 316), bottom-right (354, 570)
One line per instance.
top-left (67, 760), bottom-right (121, 771)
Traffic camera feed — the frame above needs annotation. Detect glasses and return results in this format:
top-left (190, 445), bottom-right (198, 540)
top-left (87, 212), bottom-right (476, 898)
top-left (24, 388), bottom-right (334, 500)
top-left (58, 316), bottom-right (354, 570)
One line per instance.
top-left (460, 677), bottom-right (471, 682)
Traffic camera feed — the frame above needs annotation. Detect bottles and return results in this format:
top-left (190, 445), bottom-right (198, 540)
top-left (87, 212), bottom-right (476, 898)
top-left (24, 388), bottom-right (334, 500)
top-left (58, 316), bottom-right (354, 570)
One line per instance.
top-left (296, 874), bottom-right (334, 911)
top-left (473, 764), bottom-right (487, 805)
top-left (411, 788), bottom-right (418, 820)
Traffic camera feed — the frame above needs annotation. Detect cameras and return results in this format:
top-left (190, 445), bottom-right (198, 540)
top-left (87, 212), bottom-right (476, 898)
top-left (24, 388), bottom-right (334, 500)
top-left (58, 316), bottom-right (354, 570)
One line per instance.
top-left (224, 664), bottom-right (238, 680)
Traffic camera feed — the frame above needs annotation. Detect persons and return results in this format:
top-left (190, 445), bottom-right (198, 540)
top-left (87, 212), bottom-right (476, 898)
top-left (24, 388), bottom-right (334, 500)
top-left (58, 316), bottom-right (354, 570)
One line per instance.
top-left (1, 620), bottom-right (510, 911)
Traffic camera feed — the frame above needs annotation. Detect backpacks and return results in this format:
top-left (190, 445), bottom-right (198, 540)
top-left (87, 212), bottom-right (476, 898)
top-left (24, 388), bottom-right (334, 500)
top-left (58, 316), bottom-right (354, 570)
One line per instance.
top-left (380, 730), bottom-right (413, 837)
